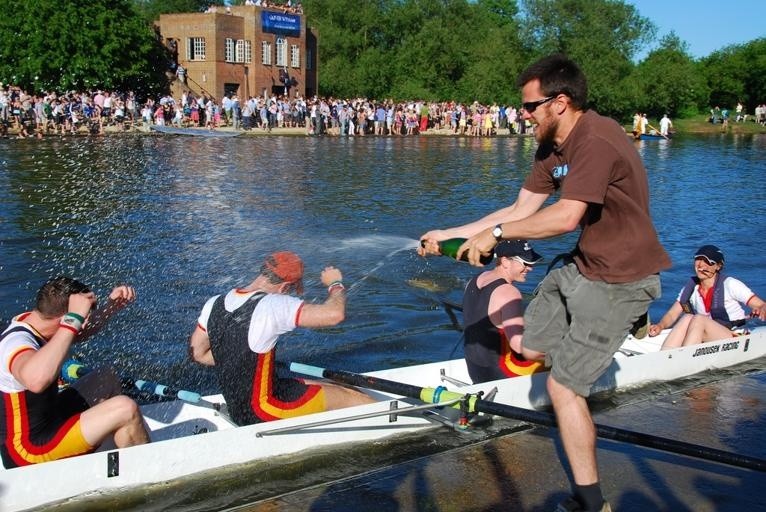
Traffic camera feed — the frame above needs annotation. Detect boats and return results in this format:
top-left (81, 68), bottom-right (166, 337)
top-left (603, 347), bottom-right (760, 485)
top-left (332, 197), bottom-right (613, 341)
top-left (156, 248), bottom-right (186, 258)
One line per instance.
top-left (0, 313), bottom-right (766, 510)
top-left (631, 125), bottom-right (676, 143)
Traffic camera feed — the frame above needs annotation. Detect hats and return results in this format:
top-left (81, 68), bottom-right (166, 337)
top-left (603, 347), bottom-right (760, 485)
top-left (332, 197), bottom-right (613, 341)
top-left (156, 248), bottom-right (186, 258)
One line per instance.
top-left (265, 252), bottom-right (304, 295)
top-left (494, 239), bottom-right (543, 264)
top-left (694, 245), bottom-right (724, 264)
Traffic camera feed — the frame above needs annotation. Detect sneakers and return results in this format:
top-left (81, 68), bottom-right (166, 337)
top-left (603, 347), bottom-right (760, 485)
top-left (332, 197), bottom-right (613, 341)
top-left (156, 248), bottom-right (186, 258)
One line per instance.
top-left (631, 312), bottom-right (649, 339)
top-left (552, 496), bottom-right (612, 512)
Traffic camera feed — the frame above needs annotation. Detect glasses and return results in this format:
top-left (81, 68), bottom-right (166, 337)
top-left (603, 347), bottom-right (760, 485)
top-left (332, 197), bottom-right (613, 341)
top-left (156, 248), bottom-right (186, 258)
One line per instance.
top-left (524, 96), bottom-right (554, 113)
top-left (513, 258), bottom-right (528, 266)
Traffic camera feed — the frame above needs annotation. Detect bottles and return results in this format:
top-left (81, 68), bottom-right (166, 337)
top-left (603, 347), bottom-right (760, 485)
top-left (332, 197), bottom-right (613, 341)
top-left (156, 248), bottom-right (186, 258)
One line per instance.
top-left (421, 239), bottom-right (493, 265)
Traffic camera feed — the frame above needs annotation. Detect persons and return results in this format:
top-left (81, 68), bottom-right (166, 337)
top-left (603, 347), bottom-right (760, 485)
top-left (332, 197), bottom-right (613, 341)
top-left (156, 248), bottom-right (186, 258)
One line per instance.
top-left (417, 54), bottom-right (673, 512)
top-left (710, 102), bottom-right (766, 127)
top-left (659, 113), bottom-right (672, 136)
top-left (648, 245), bottom-right (766, 350)
top-left (175, 65), bottom-right (185, 82)
top-left (0, 277), bottom-right (151, 470)
top-left (0, 85), bottom-right (533, 139)
top-left (188, 251), bottom-right (380, 426)
top-left (463, 239), bottom-right (546, 385)
top-left (630, 111), bottom-right (649, 136)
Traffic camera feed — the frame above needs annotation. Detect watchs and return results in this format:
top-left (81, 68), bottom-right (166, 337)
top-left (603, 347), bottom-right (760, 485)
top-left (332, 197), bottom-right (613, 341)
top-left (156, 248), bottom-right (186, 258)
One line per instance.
top-left (492, 224), bottom-right (503, 242)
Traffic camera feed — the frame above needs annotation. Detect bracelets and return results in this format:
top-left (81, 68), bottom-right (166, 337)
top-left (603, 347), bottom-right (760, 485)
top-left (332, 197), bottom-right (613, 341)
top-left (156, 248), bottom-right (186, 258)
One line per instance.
top-left (327, 281), bottom-right (346, 295)
top-left (58, 313), bottom-right (85, 335)
